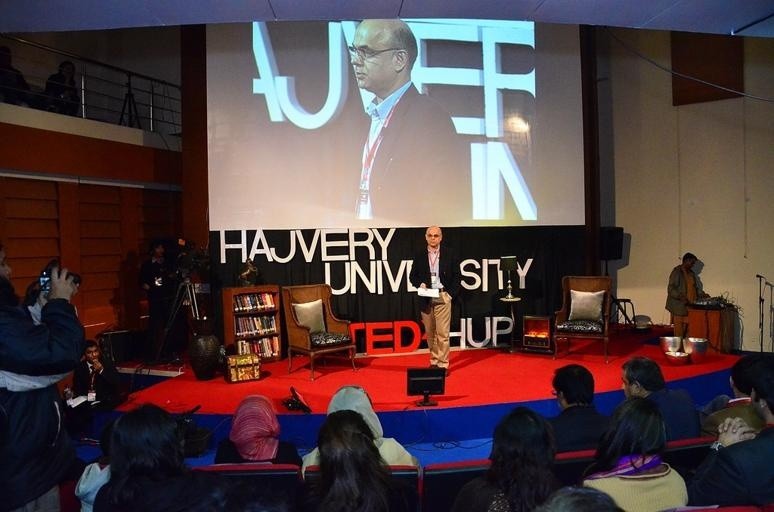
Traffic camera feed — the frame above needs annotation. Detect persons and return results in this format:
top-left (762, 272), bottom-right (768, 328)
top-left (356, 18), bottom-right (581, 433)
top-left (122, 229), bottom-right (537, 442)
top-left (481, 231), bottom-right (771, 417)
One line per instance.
top-left (76, 402), bottom-right (228, 511)
top-left (217, 385), bottom-right (404, 512)
top-left (0, 240), bottom-right (84, 512)
top-left (77, 344), bottom-right (123, 408)
top-left (138, 236), bottom-right (183, 365)
top-left (503, 352), bottom-right (774, 512)
top-left (411, 227), bottom-right (460, 376)
top-left (665, 253), bottom-right (698, 337)
top-left (334, 17), bottom-right (470, 225)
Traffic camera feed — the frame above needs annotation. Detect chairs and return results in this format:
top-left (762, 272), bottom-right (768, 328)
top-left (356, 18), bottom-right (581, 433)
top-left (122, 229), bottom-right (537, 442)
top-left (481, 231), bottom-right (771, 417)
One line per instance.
top-left (282, 284), bottom-right (357, 381)
top-left (422, 459), bottom-right (492, 511)
top-left (305, 465), bottom-right (419, 512)
top-left (192, 464), bottom-right (303, 500)
top-left (555, 448), bottom-right (598, 485)
top-left (553, 275), bottom-right (612, 356)
top-left (664, 437), bottom-right (719, 481)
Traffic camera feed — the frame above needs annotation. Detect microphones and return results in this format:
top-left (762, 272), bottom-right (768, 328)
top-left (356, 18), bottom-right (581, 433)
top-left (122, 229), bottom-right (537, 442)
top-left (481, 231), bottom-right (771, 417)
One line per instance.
top-left (756, 274), bottom-right (765, 279)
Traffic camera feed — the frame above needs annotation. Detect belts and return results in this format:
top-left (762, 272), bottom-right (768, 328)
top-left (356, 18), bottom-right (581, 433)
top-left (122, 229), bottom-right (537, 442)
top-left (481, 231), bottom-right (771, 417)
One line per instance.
top-left (439, 288), bottom-right (445, 292)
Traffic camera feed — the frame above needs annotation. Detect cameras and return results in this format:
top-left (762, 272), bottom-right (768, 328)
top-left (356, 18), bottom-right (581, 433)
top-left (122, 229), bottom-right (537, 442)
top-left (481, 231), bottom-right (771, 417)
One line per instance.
top-left (38, 261), bottom-right (81, 291)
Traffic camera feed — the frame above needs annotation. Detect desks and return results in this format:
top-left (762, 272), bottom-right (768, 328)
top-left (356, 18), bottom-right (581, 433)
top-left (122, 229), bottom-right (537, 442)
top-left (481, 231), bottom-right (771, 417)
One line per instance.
top-left (499, 297), bottom-right (520, 354)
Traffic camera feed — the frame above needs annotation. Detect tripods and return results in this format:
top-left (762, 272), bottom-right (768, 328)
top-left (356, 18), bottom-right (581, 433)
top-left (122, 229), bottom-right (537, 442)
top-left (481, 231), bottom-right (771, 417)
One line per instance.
top-left (118, 72), bottom-right (142, 130)
top-left (152, 280), bottom-right (214, 369)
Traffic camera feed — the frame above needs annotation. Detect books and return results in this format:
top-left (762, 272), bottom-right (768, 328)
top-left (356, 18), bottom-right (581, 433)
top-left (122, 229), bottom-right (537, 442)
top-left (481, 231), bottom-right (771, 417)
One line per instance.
top-left (234, 294), bottom-right (279, 358)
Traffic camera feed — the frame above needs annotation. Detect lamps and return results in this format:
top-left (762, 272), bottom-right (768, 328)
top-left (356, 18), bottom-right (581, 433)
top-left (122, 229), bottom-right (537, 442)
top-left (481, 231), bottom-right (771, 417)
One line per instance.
top-left (500, 255), bottom-right (518, 298)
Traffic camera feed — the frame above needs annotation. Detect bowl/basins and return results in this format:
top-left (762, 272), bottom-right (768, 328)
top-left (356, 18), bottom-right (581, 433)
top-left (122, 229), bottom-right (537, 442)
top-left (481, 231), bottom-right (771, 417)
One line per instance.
top-left (665, 352), bottom-right (689, 366)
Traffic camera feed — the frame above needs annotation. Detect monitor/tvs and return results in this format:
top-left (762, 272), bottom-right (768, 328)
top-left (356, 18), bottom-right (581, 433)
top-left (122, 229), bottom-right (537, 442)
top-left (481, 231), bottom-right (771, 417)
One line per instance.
top-left (407, 368), bottom-right (446, 407)
top-left (285, 386), bottom-right (311, 413)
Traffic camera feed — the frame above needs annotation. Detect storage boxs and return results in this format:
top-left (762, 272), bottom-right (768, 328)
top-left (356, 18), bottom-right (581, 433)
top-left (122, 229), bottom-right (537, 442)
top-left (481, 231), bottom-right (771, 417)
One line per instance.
top-left (523, 314), bottom-right (552, 354)
top-left (225, 354), bottom-right (261, 381)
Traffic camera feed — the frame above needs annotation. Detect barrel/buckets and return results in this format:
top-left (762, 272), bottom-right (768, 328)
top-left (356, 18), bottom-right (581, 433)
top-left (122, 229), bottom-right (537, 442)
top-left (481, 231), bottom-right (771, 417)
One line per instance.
top-left (660, 336), bottom-right (682, 358)
top-left (682, 337), bottom-right (707, 362)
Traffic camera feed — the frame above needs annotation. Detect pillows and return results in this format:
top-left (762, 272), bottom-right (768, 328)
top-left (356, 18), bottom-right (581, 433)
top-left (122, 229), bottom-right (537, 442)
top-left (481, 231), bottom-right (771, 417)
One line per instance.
top-left (292, 298), bottom-right (327, 334)
top-left (569, 289), bottom-right (605, 323)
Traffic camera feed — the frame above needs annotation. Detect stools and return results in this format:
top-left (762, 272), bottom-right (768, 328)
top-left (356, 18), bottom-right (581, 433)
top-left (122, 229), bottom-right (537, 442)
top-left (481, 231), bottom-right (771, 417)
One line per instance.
top-left (611, 298), bottom-right (636, 328)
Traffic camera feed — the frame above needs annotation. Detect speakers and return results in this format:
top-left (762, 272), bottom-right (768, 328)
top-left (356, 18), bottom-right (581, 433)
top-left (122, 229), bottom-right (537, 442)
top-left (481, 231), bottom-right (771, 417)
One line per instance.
top-left (600, 227), bottom-right (623, 259)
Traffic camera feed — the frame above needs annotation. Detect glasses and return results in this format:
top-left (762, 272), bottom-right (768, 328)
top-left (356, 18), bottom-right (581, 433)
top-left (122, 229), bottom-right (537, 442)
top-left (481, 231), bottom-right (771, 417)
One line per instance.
top-left (349, 46), bottom-right (400, 59)
top-left (552, 391), bottom-right (558, 395)
top-left (426, 234), bottom-right (439, 237)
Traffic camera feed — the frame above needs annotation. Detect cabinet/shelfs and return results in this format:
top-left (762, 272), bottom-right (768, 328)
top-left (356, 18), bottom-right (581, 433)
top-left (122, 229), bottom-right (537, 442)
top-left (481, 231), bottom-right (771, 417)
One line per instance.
top-left (222, 285), bottom-right (282, 359)
top-left (687, 308), bottom-right (735, 352)
